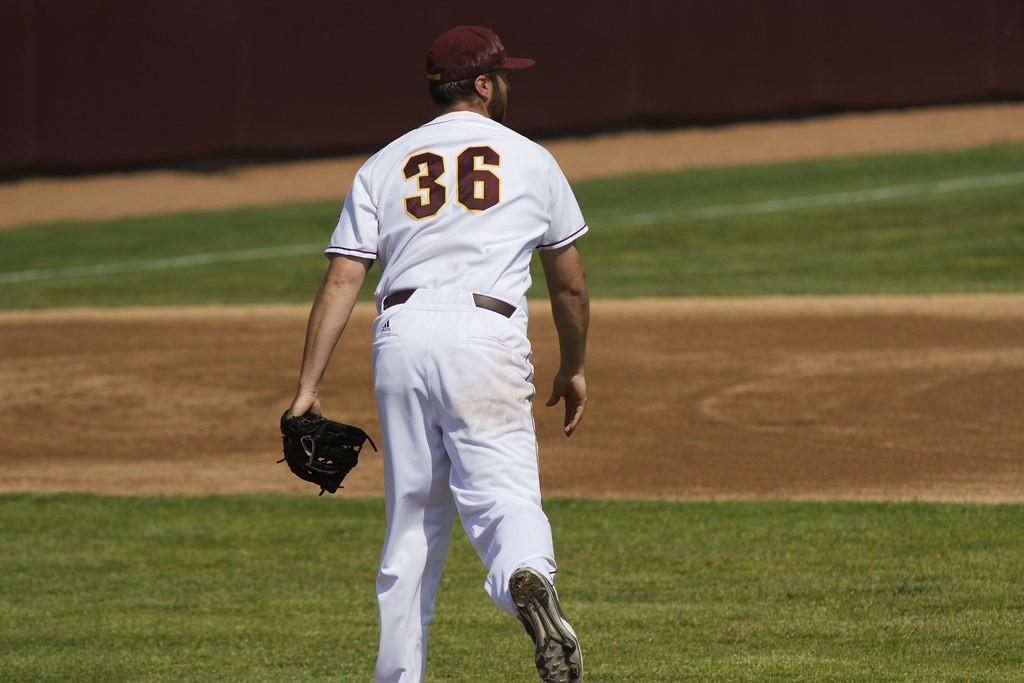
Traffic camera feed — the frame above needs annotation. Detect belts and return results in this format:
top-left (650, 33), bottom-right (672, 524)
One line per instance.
top-left (384, 289), bottom-right (517, 318)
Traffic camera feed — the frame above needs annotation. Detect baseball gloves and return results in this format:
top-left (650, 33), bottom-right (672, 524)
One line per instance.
top-left (276, 408), bottom-right (380, 497)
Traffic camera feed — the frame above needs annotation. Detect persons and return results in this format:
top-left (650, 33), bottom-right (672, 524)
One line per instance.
top-left (286, 25), bottom-right (590, 683)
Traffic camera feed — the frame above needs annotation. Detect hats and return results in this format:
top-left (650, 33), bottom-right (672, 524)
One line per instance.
top-left (425, 26), bottom-right (537, 83)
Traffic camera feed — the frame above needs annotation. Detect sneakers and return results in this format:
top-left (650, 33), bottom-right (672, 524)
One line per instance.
top-left (509, 567), bottom-right (583, 683)
